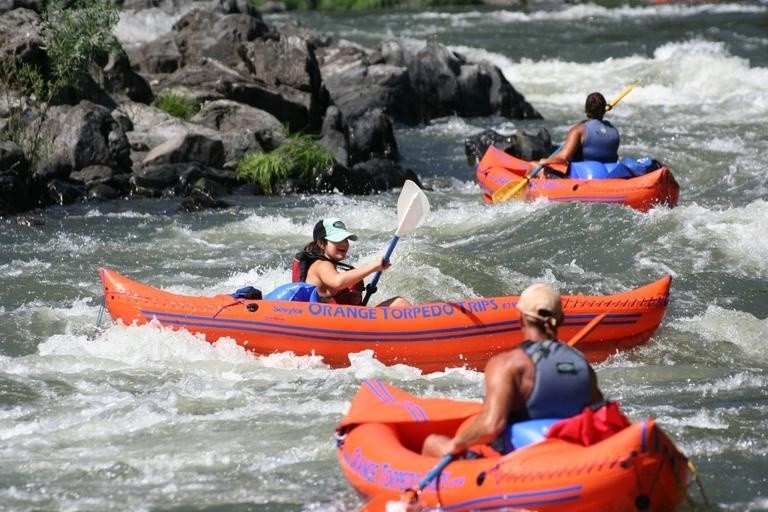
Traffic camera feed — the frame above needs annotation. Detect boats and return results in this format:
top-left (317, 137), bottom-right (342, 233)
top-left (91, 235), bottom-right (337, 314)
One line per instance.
top-left (335, 377), bottom-right (694, 512)
top-left (96, 266), bottom-right (672, 373)
top-left (475, 144), bottom-right (680, 213)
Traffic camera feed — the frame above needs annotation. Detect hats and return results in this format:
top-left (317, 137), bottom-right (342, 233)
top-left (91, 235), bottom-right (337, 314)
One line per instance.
top-left (514, 283), bottom-right (562, 327)
top-left (313, 217), bottom-right (358, 244)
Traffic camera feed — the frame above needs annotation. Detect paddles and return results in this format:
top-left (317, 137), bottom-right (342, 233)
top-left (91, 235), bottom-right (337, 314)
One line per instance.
top-left (359, 310), bottom-right (609, 512)
top-left (492, 86), bottom-right (634, 204)
top-left (361, 179), bottom-right (431, 305)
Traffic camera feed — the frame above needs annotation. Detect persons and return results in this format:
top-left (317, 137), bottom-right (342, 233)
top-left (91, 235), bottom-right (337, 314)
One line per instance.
top-left (538, 92), bottom-right (621, 165)
top-left (422, 284), bottom-right (604, 459)
top-left (306, 218), bottom-right (412, 306)
